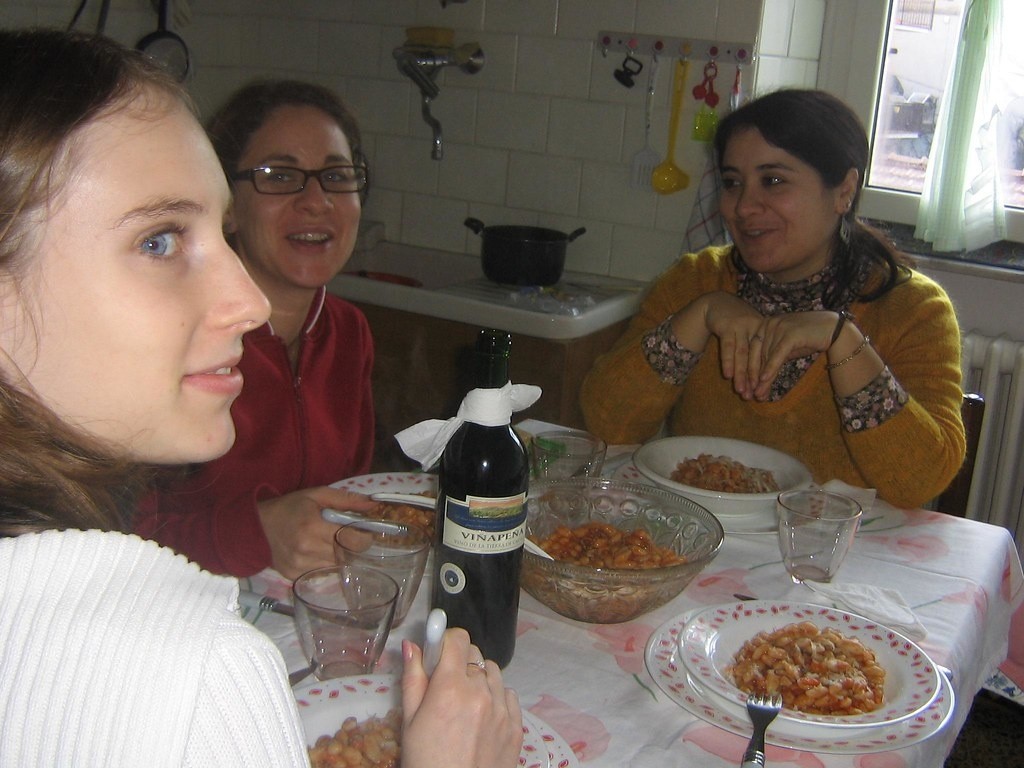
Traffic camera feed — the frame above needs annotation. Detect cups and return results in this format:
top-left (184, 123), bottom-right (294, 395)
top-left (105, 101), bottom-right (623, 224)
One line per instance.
top-left (291, 566), bottom-right (400, 682)
top-left (531, 430), bottom-right (607, 482)
top-left (776, 489), bottom-right (862, 587)
top-left (334, 520), bottom-right (433, 629)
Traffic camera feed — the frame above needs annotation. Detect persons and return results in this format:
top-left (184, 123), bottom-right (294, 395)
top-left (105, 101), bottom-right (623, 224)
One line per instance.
top-left (577, 88), bottom-right (970, 508)
top-left (122, 76), bottom-right (378, 582)
top-left (1, 27), bottom-right (527, 768)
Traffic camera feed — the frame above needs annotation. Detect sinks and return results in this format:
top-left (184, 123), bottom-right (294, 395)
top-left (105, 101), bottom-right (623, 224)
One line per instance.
top-left (339, 246), bottom-right (481, 289)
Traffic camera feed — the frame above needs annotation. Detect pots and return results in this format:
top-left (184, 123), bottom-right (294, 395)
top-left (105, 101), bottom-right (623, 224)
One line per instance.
top-left (463, 217), bottom-right (587, 290)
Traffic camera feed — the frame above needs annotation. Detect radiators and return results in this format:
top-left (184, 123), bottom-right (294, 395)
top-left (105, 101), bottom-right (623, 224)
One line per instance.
top-left (926, 333), bottom-right (1024, 541)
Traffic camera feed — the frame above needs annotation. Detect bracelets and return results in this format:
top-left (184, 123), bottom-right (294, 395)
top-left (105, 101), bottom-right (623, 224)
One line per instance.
top-left (829, 310), bottom-right (856, 344)
top-left (825, 335), bottom-right (869, 369)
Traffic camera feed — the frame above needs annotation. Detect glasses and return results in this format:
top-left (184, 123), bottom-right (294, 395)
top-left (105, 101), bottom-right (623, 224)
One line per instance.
top-left (230, 165), bottom-right (370, 196)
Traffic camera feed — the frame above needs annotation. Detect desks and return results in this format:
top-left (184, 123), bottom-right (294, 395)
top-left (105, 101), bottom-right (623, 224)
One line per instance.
top-left (231, 413), bottom-right (1024, 768)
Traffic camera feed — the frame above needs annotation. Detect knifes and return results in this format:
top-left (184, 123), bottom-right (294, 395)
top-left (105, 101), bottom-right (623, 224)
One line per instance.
top-left (732, 592), bottom-right (952, 601)
top-left (288, 668), bottom-right (311, 687)
top-left (238, 590), bottom-right (375, 631)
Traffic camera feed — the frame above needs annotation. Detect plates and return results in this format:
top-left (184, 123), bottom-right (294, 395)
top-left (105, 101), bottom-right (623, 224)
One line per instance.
top-left (677, 601), bottom-right (940, 729)
top-left (611, 460), bottom-right (827, 536)
top-left (644, 603), bottom-right (956, 755)
top-left (293, 674), bottom-right (582, 768)
top-left (321, 471), bottom-right (441, 577)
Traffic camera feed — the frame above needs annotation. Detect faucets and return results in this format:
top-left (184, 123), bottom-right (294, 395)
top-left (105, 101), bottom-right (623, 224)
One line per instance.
top-left (392, 46), bottom-right (444, 161)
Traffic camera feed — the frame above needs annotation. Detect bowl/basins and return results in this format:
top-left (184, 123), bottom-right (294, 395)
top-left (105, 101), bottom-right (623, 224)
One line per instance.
top-left (343, 271), bottom-right (423, 289)
top-left (631, 435), bottom-right (810, 519)
top-left (516, 478), bottom-right (724, 624)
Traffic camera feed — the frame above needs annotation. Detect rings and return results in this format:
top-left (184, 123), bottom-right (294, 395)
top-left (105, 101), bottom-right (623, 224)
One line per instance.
top-left (468, 661), bottom-right (487, 677)
top-left (754, 333), bottom-right (763, 342)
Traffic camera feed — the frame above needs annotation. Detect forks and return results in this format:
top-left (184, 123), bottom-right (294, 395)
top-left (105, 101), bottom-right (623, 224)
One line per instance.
top-left (740, 691), bottom-right (783, 768)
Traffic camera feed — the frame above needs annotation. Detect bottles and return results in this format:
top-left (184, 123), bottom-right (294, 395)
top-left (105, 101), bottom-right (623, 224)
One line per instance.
top-left (433, 328), bottom-right (529, 671)
top-left (357, 269), bottom-right (367, 277)
